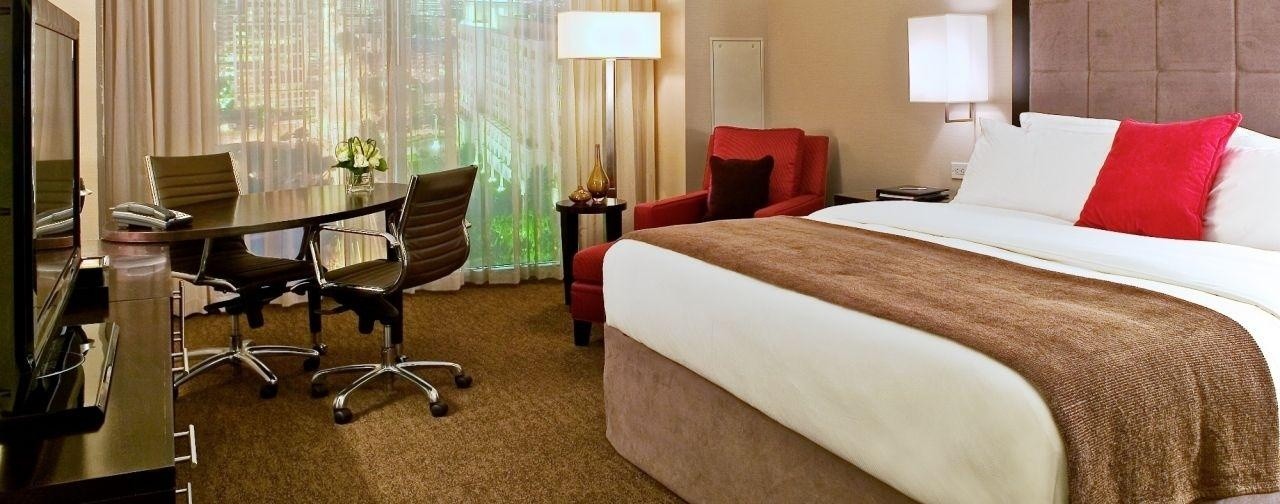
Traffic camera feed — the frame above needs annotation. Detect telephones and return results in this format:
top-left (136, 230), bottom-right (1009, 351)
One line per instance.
top-left (109, 201), bottom-right (192, 230)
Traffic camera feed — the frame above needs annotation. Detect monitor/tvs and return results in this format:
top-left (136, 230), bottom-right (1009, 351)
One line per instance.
top-left (13, 0), bottom-right (121, 435)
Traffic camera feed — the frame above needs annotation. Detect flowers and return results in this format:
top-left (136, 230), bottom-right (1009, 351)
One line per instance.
top-left (330, 136), bottom-right (388, 183)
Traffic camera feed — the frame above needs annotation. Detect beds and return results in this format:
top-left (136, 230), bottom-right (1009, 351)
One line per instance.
top-left (603, 0), bottom-right (1280, 504)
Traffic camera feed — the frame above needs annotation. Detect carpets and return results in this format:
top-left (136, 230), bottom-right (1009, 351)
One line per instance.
top-left (174, 280), bottom-right (693, 504)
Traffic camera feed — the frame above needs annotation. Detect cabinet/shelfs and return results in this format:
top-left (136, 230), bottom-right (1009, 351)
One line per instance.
top-left (0, 0), bottom-right (197, 504)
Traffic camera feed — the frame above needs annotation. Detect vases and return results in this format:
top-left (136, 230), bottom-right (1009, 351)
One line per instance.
top-left (346, 172), bottom-right (375, 197)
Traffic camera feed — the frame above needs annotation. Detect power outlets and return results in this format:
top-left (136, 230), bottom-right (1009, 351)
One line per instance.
top-left (951, 162), bottom-right (968, 179)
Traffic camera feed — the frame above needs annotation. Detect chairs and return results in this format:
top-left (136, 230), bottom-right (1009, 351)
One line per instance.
top-left (145, 153), bottom-right (321, 399)
top-left (313, 164), bottom-right (473, 425)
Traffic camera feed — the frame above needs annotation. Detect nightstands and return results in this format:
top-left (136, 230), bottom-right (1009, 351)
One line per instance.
top-left (834, 190), bottom-right (954, 203)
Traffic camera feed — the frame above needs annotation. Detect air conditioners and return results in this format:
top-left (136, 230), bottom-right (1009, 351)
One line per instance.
top-left (709, 35), bottom-right (763, 129)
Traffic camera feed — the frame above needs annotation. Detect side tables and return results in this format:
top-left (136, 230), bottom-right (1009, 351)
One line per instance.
top-left (556, 191), bottom-right (626, 307)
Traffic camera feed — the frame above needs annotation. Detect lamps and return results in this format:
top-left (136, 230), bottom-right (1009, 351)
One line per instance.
top-left (910, 15), bottom-right (987, 134)
top-left (559, 11), bottom-right (661, 167)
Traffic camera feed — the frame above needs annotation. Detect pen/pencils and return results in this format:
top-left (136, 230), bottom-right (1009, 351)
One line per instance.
top-left (899, 187), bottom-right (928, 190)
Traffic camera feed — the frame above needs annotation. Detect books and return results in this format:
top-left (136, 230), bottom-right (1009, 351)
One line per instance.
top-left (875, 184), bottom-right (950, 201)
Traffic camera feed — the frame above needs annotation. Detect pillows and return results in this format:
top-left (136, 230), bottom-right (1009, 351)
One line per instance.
top-left (707, 154), bottom-right (773, 220)
top-left (955, 110), bottom-right (1118, 219)
top-left (1202, 146), bottom-right (1280, 251)
top-left (1074, 113), bottom-right (1241, 240)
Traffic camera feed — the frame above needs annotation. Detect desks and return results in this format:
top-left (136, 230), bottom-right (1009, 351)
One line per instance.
top-left (102, 180), bottom-right (438, 369)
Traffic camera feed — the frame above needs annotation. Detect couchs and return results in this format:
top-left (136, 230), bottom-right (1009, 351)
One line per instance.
top-left (570, 126), bottom-right (828, 347)
